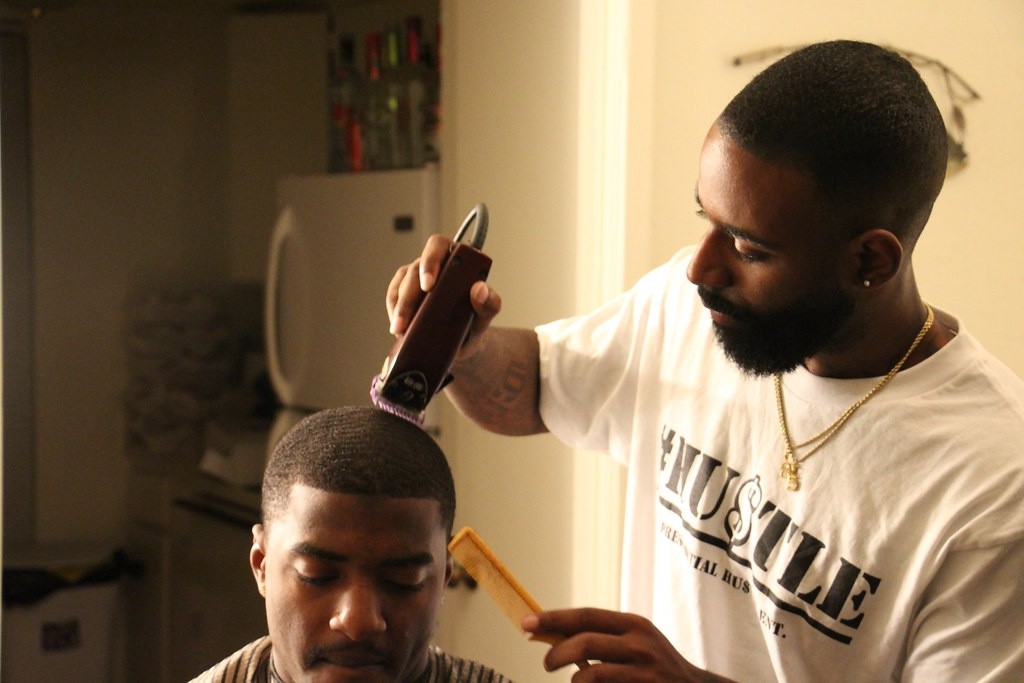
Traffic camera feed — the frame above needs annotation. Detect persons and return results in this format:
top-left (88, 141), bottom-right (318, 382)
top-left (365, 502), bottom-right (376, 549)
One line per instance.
top-left (184, 406), bottom-right (512, 683)
top-left (383, 38), bottom-right (1022, 683)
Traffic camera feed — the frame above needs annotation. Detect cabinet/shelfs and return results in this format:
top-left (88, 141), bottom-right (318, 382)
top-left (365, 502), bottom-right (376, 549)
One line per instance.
top-left (124, 461), bottom-right (273, 682)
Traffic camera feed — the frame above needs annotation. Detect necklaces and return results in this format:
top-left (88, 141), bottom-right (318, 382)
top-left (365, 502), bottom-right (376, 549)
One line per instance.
top-left (774, 303), bottom-right (935, 491)
